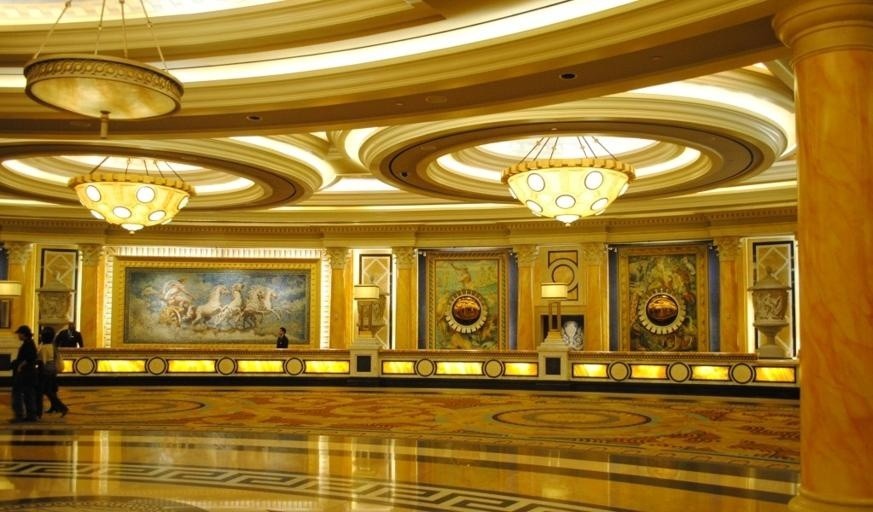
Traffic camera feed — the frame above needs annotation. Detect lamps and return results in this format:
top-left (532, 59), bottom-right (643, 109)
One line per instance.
top-left (0, 280), bottom-right (21, 328)
top-left (501, 134), bottom-right (635, 227)
top-left (23, 1), bottom-right (183, 141)
top-left (540, 282), bottom-right (567, 331)
top-left (353, 285), bottom-right (378, 337)
top-left (67, 155), bottom-right (194, 234)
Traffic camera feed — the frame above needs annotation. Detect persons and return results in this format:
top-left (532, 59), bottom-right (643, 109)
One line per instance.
top-left (36, 329), bottom-right (68, 419)
top-left (39, 327), bottom-right (58, 413)
top-left (54, 321), bottom-right (83, 347)
top-left (275, 327), bottom-right (288, 349)
top-left (7, 324), bottom-right (38, 422)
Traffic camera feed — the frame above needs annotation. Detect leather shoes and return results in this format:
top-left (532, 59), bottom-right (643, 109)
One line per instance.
top-left (62, 405), bottom-right (68, 416)
top-left (47, 407), bottom-right (58, 412)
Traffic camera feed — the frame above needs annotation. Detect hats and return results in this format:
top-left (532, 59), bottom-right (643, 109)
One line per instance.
top-left (15, 326), bottom-right (30, 334)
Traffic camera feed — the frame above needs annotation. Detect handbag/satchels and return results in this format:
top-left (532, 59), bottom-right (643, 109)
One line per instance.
top-left (43, 361), bottom-right (56, 372)
